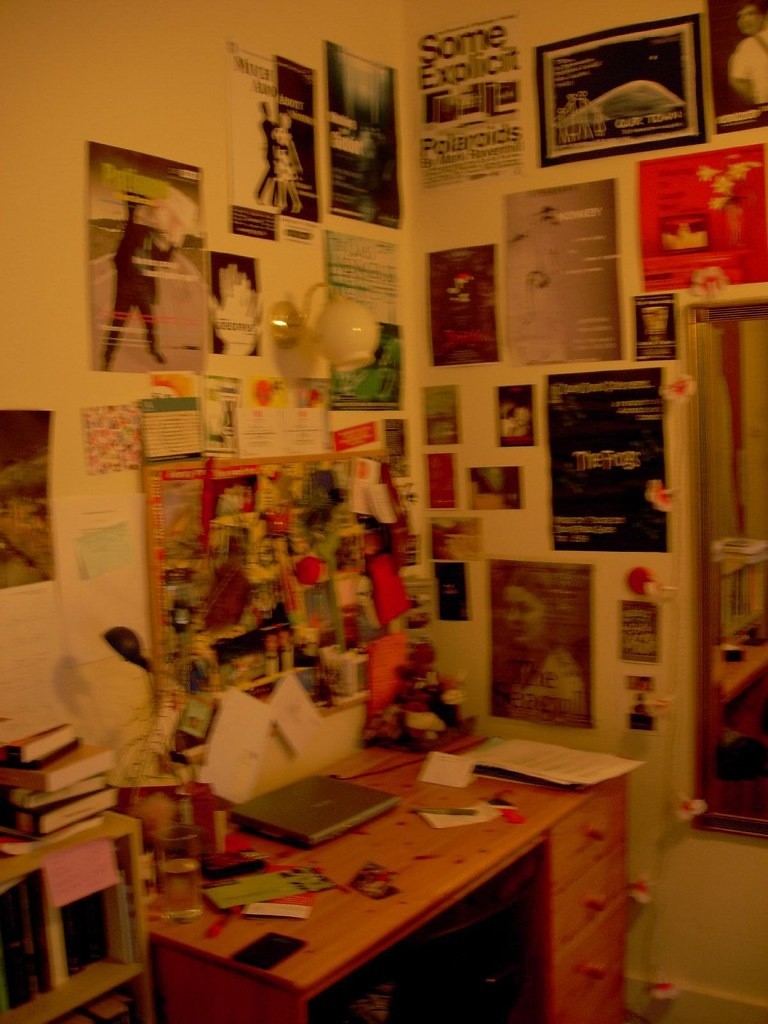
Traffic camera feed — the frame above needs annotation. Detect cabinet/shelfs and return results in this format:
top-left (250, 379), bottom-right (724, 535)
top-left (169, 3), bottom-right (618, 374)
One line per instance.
top-left (151, 739), bottom-right (630, 1024)
top-left (0, 808), bottom-right (154, 1024)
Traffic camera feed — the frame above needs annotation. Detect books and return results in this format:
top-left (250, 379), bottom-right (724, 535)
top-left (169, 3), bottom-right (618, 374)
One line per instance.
top-left (0, 716), bottom-right (117, 855)
top-left (0, 856), bottom-right (138, 1024)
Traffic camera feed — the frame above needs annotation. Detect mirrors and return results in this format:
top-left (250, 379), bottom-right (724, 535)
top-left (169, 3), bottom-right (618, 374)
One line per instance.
top-left (687, 300), bottom-right (768, 839)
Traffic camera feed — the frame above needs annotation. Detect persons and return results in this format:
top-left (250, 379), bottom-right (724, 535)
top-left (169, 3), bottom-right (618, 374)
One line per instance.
top-left (729, 5), bottom-right (768, 112)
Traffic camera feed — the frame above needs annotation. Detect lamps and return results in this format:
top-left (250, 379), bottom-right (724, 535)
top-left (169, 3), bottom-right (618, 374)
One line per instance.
top-left (270, 282), bottom-right (383, 374)
top-left (98, 625), bottom-right (151, 673)
